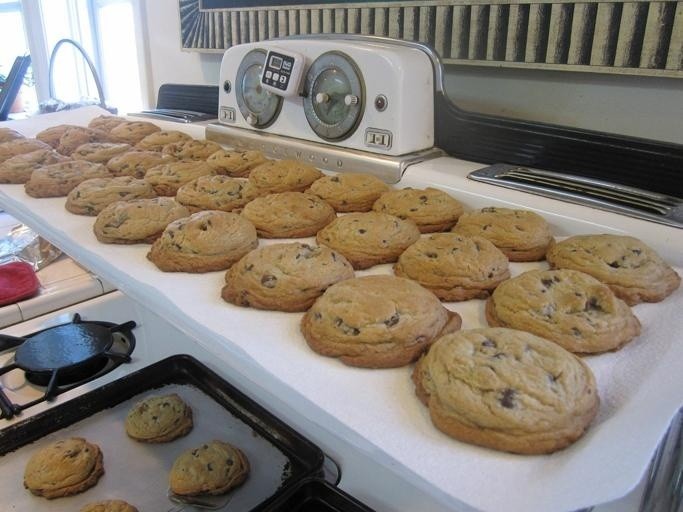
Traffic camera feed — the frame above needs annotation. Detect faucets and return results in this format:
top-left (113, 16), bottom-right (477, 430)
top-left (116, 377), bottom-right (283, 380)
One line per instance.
top-left (38, 37), bottom-right (119, 116)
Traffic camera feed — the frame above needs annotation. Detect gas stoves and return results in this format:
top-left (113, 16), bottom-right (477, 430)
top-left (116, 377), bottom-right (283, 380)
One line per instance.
top-left (1, 312), bottom-right (137, 421)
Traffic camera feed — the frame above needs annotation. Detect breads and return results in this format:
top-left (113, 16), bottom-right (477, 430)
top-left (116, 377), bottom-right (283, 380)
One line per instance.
top-left (136, 129), bottom-right (191, 152)
top-left (36, 124), bottom-right (78, 147)
top-left (93, 196), bottom-right (191, 242)
top-left (79, 499), bottom-right (136, 512)
top-left (309, 172), bottom-right (386, 211)
top-left (57, 125), bottom-right (103, 154)
top-left (23, 160), bottom-right (107, 197)
top-left (220, 241), bottom-right (353, 312)
top-left (22, 438), bottom-right (104, 501)
top-left (488, 269), bottom-right (642, 357)
top-left (105, 150), bottom-right (178, 177)
top-left (301, 273), bottom-right (461, 368)
top-left (250, 157), bottom-right (325, 196)
top-left (88, 114), bottom-right (126, 134)
top-left (455, 206), bottom-right (557, 260)
top-left (163, 138), bottom-right (222, 163)
top-left (126, 394), bottom-right (193, 445)
top-left (176, 173), bottom-right (254, 211)
top-left (0, 126), bottom-right (24, 144)
top-left (394, 232), bottom-right (511, 300)
top-left (65, 175), bottom-right (151, 216)
top-left (374, 186), bottom-right (465, 233)
top-left (241, 190), bottom-right (338, 240)
top-left (72, 141), bottom-right (130, 163)
top-left (415, 328), bottom-right (600, 454)
top-left (110, 120), bottom-right (159, 144)
top-left (169, 441), bottom-right (249, 498)
top-left (547, 233), bottom-right (681, 304)
top-left (146, 160), bottom-right (223, 195)
top-left (147, 209), bottom-right (257, 273)
top-left (0, 150), bottom-right (53, 184)
top-left (0, 137), bottom-right (55, 162)
top-left (205, 150), bottom-right (272, 176)
top-left (316, 212), bottom-right (419, 269)
